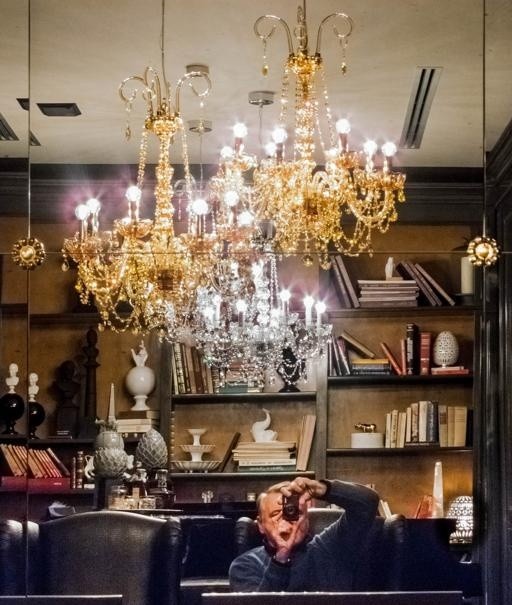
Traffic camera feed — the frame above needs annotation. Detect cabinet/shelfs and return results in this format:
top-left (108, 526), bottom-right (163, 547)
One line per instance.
top-left (29, 164), bottom-right (484, 547)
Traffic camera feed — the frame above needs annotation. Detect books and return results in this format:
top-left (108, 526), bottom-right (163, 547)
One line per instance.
top-left (328, 322), bottom-right (471, 375)
top-left (384, 400), bottom-right (473, 448)
top-left (171, 339), bottom-right (218, 396)
top-left (28, 444), bottom-right (71, 479)
top-left (0, 444), bottom-right (27, 477)
top-left (217, 414), bottom-right (317, 472)
top-left (328, 254), bottom-right (456, 307)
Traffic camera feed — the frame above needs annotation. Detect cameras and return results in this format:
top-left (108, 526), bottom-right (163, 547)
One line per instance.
top-left (281, 493), bottom-right (300, 522)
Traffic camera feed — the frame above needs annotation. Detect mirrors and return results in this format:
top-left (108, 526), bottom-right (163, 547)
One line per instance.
top-left (1, 0), bottom-right (511, 604)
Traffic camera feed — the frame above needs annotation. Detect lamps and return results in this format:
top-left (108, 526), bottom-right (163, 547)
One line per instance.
top-left (252, 3), bottom-right (406, 271)
top-left (60, 64), bottom-right (332, 390)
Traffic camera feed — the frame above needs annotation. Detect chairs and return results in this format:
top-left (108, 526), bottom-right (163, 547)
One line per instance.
top-left (25, 509), bottom-right (182, 604)
top-left (305, 507), bottom-right (408, 591)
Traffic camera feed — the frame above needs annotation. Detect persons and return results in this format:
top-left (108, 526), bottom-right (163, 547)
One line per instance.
top-left (228, 477), bottom-right (379, 593)
top-left (46, 361), bottom-right (82, 408)
top-left (5, 363), bottom-right (20, 395)
top-left (28, 372), bottom-right (40, 402)
